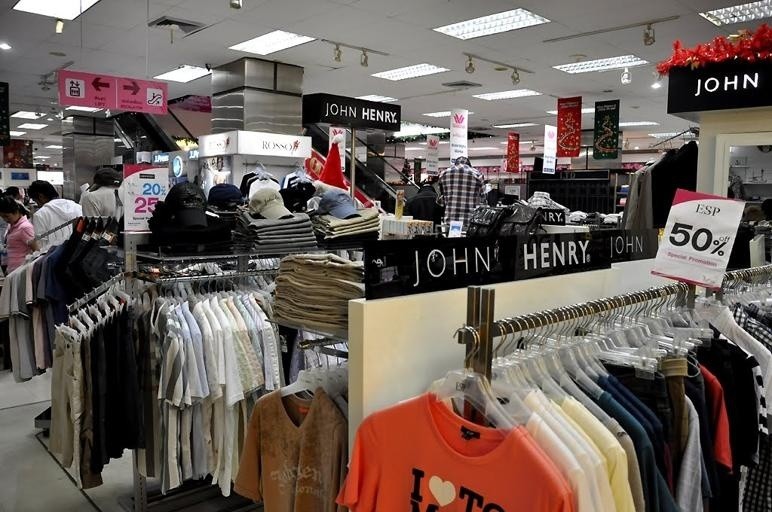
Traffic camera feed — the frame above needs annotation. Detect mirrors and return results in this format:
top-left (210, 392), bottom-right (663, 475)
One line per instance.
top-left (715, 133), bottom-right (771, 268)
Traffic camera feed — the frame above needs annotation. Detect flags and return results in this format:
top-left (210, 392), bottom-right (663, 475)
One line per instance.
top-left (505, 132), bottom-right (520, 173)
top-left (541, 124), bottom-right (558, 175)
top-left (3, 139), bottom-right (35, 170)
top-left (556, 96), bottom-right (582, 157)
top-left (0, 81), bottom-right (11, 147)
top-left (591, 99), bottom-right (620, 160)
top-left (449, 108), bottom-right (469, 165)
top-left (328, 127), bottom-right (347, 173)
top-left (426, 135), bottom-right (439, 173)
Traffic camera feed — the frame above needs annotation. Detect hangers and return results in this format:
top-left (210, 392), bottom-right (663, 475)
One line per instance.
top-left (427, 265), bottom-right (772, 438)
top-left (158, 272), bottom-right (348, 417)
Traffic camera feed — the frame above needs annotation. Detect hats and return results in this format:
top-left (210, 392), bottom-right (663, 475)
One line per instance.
top-left (164, 182), bottom-right (361, 219)
top-left (744, 199), bottom-right (772, 221)
top-left (89, 168), bottom-right (119, 192)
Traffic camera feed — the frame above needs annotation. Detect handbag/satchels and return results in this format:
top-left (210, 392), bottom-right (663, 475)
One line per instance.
top-left (466, 206), bottom-right (547, 237)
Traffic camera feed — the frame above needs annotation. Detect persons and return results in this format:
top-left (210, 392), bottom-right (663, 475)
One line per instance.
top-left (436, 156), bottom-right (487, 228)
top-left (0, 197), bottom-right (36, 278)
top-left (27, 179), bottom-right (84, 254)
top-left (0, 186), bottom-right (23, 277)
top-left (404, 183), bottom-right (445, 226)
top-left (81, 166), bottom-right (125, 225)
